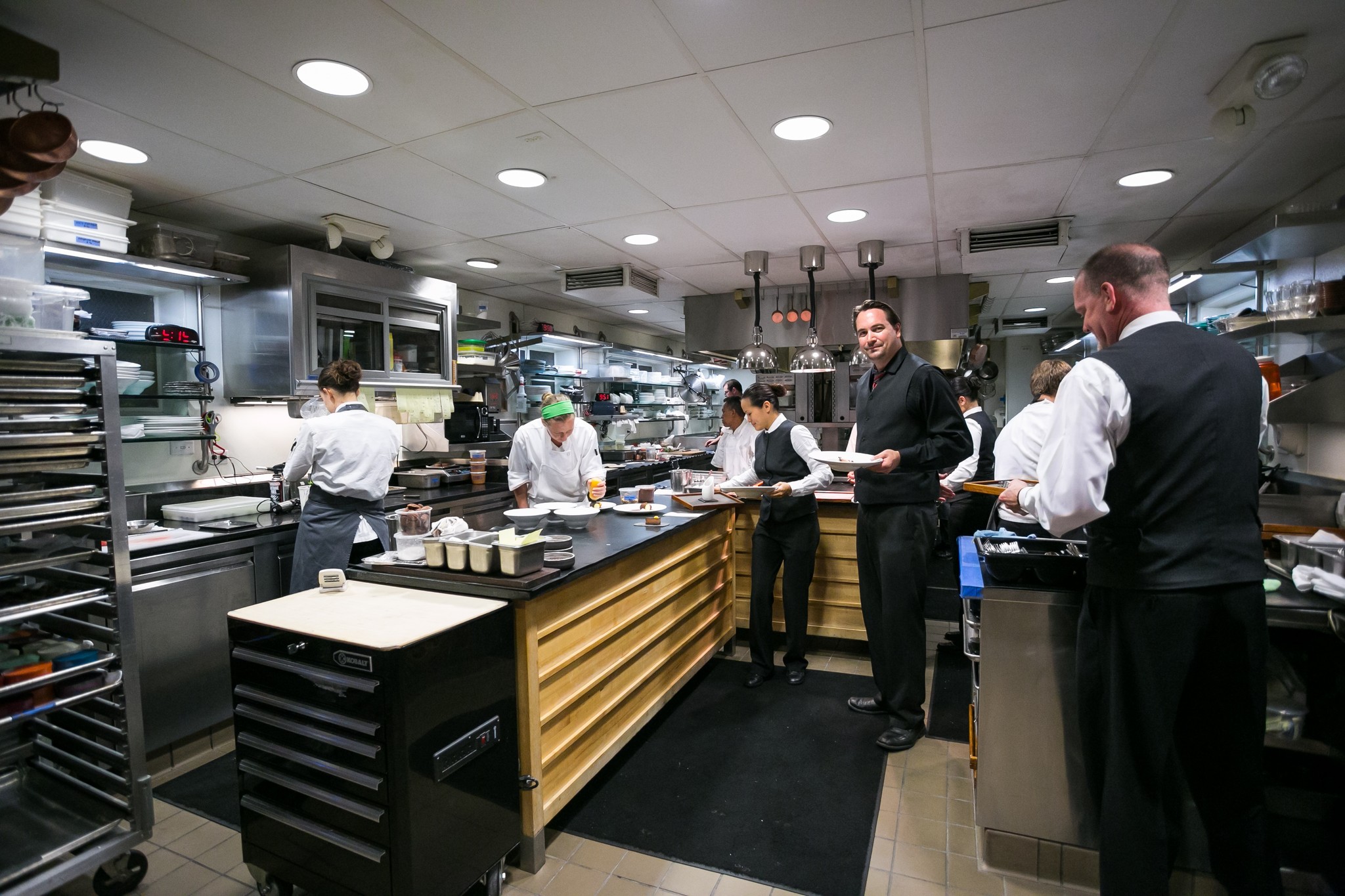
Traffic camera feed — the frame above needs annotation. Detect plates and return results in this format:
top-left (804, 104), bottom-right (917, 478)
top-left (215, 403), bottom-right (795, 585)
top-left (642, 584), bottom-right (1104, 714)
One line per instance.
top-left (575, 502), bottom-right (668, 512)
top-left (504, 500), bottom-right (599, 531)
top-left (720, 484), bottom-right (777, 500)
top-left (808, 445), bottom-right (884, 473)
top-left (524, 384), bottom-right (552, 420)
top-left (622, 367), bottom-right (682, 403)
top-left (521, 358), bottom-right (587, 377)
top-left (112, 320), bottom-right (205, 435)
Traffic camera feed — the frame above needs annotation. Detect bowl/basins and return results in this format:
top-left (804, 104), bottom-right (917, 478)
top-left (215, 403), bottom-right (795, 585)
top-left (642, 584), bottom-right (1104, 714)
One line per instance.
top-left (127, 518), bottom-right (160, 534)
top-left (544, 534), bottom-right (575, 571)
top-left (468, 450), bottom-right (487, 485)
top-left (560, 384), bottom-right (583, 402)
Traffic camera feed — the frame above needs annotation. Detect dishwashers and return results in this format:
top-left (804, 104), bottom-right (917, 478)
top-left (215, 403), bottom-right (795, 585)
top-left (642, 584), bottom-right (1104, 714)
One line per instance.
top-left (123, 551), bottom-right (258, 756)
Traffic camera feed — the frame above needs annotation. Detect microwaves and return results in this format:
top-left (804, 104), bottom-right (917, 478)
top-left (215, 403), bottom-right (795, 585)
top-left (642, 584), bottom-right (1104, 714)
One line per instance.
top-left (443, 401), bottom-right (490, 444)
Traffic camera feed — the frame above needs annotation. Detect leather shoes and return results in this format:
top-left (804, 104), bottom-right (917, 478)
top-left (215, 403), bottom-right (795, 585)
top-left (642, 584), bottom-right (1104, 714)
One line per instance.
top-left (847, 697), bottom-right (888, 714)
top-left (743, 667), bottom-right (775, 687)
top-left (786, 668), bottom-right (806, 685)
top-left (876, 721), bottom-right (926, 750)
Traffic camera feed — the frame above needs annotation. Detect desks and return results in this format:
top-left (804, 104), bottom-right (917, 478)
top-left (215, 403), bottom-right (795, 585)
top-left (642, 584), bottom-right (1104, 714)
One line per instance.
top-left (345, 497), bottom-right (737, 875)
top-left (648, 478), bottom-right (932, 643)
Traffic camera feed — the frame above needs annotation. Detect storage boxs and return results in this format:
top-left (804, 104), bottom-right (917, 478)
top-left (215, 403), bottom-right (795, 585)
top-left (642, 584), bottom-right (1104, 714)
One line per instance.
top-left (393, 507), bottom-right (553, 578)
top-left (160, 496), bottom-right (271, 523)
top-left (458, 339), bottom-right (498, 367)
top-left (128, 221), bottom-right (250, 274)
top-left (1260, 494), bottom-right (1344, 578)
top-left (0, 166), bottom-right (138, 329)
top-left (469, 450), bottom-right (486, 462)
top-left (393, 470), bottom-right (444, 489)
top-left (975, 535), bottom-right (1090, 588)
top-left (465, 300), bottom-right (489, 320)
top-left (470, 471), bottom-right (487, 484)
top-left (779, 390), bottom-right (793, 407)
top-left (469, 461), bottom-right (487, 472)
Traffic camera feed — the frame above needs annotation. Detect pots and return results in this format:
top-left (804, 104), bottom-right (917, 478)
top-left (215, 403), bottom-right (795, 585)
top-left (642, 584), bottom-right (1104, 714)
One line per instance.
top-left (669, 468), bottom-right (693, 493)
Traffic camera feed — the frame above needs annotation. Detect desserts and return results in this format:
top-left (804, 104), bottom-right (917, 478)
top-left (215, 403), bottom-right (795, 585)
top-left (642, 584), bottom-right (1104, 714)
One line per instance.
top-left (590, 500), bottom-right (602, 508)
top-left (640, 502), bottom-right (652, 511)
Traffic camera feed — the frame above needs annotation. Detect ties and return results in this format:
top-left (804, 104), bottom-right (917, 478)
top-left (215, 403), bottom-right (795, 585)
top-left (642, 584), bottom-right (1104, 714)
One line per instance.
top-left (872, 372), bottom-right (885, 392)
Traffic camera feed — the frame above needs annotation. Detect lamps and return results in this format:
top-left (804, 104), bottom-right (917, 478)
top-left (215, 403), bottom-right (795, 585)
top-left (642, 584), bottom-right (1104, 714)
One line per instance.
top-left (320, 214), bottom-right (395, 260)
top-left (466, 258), bottom-right (499, 268)
top-left (735, 240), bottom-right (885, 373)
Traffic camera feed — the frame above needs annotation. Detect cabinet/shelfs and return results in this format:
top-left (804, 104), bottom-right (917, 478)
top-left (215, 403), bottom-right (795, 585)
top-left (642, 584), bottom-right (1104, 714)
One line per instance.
top-left (220, 244), bottom-right (462, 407)
top-left (1168, 209), bottom-right (1344, 425)
top-left (43, 241), bottom-right (250, 475)
top-left (494, 331), bottom-right (733, 443)
top-left (0, 331), bottom-right (155, 896)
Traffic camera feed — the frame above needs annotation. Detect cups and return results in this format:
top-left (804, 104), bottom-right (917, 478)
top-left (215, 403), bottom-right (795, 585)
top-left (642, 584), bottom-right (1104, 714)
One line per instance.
top-left (1265, 279), bottom-right (1322, 320)
top-left (614, 435), bottom-right (625, 449)
top-left (646, 447), bottom-right (656, 461)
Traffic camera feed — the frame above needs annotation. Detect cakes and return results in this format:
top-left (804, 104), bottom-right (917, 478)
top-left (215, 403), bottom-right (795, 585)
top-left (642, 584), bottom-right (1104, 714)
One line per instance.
top-left (645, 515), bottom-right (661, 525)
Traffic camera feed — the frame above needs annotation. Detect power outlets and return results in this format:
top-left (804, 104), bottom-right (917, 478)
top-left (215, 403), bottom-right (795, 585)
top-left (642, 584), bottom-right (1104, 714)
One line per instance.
top-left (208, 449), bottom-right (229, 464)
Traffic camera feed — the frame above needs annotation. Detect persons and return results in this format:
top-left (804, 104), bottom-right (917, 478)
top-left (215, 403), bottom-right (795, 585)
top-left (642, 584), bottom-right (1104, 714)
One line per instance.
top-left (844, 422), bottom-right (858, 485)
top-left (505, 392), bottom-right (606, 509)
top-left (704, 379), bottom-right (747, 448)
top-left (937, 374), bottom-right (997, 540)
top-left (713, 382), bottom-right (834, 685)
top-left (848, 299), bottom-right (974, 756)
top-left (711, 396), bottom-right (763, 481)
top-left (281, 360), bottom-right (404, 593)
top-left (998, 241), bottom-right (1280, 896)
top-left (994, 359), bottom-right (1073, 540)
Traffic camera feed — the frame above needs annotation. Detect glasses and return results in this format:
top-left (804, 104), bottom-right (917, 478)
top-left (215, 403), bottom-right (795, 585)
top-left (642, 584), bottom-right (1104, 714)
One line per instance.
top-left (724, 386), bottom-right (734, 395)
